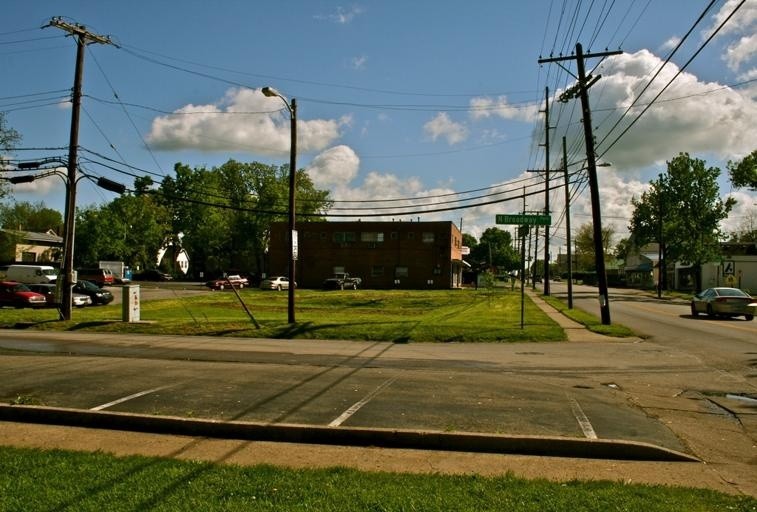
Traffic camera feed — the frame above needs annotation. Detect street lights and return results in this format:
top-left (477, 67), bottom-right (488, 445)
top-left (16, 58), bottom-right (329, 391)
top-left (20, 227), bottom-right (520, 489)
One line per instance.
top-left (563, 136), bottom-right (612, 309)
top-left (262, 87), bottom-right (296, 324)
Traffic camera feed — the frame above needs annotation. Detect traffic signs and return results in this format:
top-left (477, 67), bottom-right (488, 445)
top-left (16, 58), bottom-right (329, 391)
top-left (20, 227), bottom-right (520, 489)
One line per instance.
top-left (518, 225), bottom-right (529, 238)
top-left (496, 214), bottom-right (551, 225)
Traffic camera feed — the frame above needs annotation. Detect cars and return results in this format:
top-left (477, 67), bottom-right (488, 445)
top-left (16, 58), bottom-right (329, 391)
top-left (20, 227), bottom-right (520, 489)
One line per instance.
top-left (0, 279), bottom-right (113, 309)
top-left (206, 274), bottom-right (249, 290)
top-left (74, 268), bottom-right (173, 288)
top-left (261, 276), bottom-right (298, 292)
top-left (691, 288), bottom-right (757, 321)
top-left (554, 276), bottom-right (560, 281)
top-left (532, 274), bottom-right (542, 283)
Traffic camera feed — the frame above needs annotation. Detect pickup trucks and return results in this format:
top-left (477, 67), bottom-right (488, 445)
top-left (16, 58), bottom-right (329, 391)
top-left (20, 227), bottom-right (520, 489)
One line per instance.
top-left (323, 272), bottom-right (362, 291)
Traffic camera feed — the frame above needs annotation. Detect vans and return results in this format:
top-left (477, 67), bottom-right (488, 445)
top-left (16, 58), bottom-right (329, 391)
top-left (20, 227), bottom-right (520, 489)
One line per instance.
top-left (6, 265), bottom-right (58, 285)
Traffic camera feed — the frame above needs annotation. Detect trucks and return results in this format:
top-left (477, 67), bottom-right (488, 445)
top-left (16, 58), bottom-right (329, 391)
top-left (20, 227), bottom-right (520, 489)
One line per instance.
top-left (99, 261), bottom-right (133, 280)
